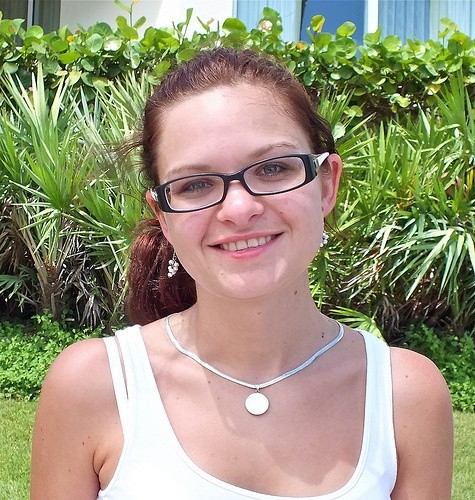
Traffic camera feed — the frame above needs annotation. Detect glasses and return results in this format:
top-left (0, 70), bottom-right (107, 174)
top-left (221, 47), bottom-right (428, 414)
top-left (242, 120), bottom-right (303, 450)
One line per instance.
top-left (149, 153), bottom-right (328, 213)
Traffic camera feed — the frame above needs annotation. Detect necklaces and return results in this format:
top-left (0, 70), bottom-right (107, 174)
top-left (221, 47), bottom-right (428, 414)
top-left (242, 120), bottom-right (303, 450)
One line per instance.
top-left (162, 312), bottom-right (346, 418)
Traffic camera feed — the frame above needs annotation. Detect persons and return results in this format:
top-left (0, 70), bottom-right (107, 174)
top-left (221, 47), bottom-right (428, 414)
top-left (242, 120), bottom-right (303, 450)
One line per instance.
top-left (31, 50), bottom-right (453, 500)
